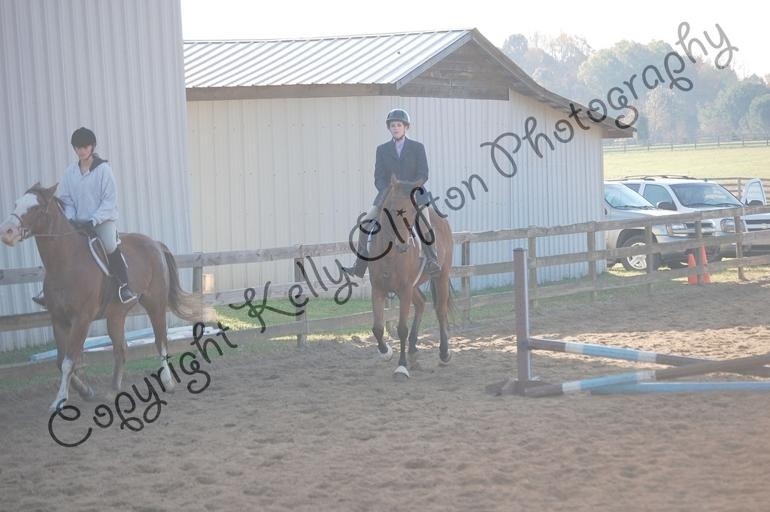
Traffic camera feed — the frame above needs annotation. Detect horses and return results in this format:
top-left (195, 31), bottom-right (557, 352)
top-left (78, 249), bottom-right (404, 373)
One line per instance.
top-left (0, 180), bottom-right (205, 413)
top-left (368, 172), bottom-right (460, 382)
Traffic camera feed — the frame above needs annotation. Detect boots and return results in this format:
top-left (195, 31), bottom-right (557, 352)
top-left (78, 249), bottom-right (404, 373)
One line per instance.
top-left (107, 247), bottom-right (133, 300)
top-left (422, 230), bottom-right (441, 272)
top-left (342, 234), bottom-right (369, 278)
top-left (32, 291), bottom-right (45, 305)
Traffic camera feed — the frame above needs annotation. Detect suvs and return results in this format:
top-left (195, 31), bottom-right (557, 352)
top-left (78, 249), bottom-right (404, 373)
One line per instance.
top-left (603, 179), bottom-right (690, 276)
top-left (619, 169), bottom-right (769, 262)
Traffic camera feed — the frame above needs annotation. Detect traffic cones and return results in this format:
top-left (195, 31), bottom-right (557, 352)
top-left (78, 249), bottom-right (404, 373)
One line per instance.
top-left (700, 245), bottom-right (712, 283)
top-left (684, 253), bottom-right (698, 285)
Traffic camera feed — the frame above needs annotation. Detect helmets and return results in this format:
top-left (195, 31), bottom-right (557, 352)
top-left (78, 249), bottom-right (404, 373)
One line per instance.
top-left (71, 128), bottom-right (96, 146)
top-left (385, 109), bottom-right (410, 128)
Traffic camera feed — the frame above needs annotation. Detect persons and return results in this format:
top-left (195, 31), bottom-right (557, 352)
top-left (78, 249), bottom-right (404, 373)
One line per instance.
top-left (340, 107), bottom-right (442, 279)
top-left (31, 126), bottom-right (136, 305)
